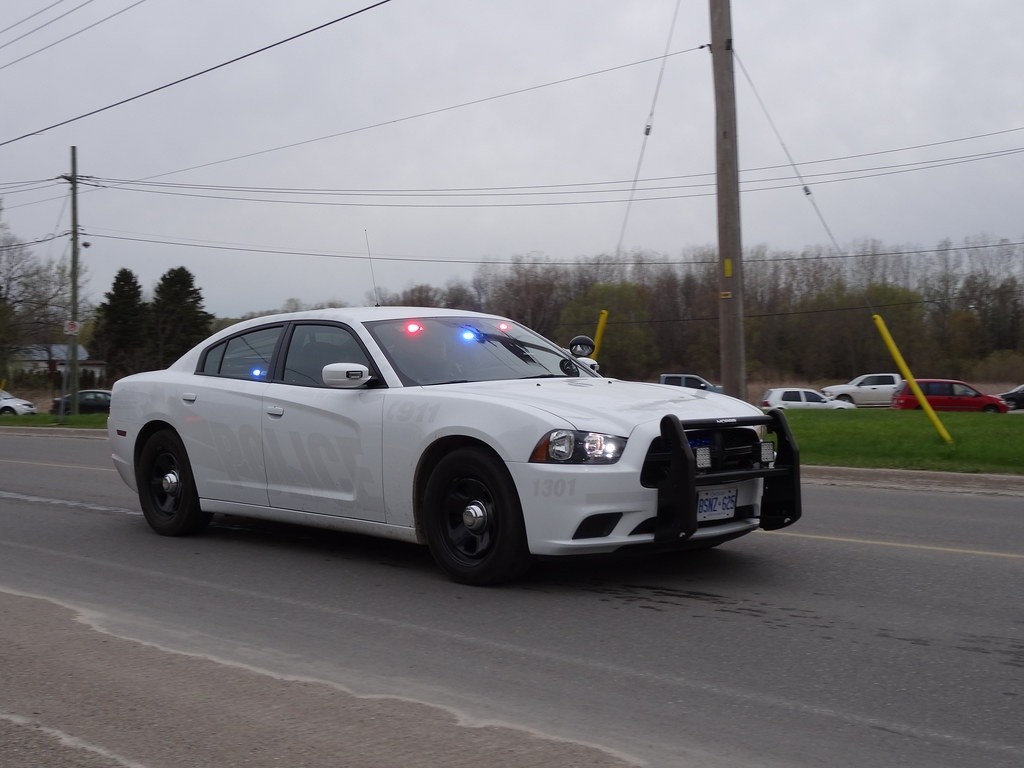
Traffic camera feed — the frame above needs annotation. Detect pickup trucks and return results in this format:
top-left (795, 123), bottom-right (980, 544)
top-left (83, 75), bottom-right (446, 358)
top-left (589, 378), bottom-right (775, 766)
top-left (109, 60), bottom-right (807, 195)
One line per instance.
top-left (820, 371), bottom-right (901, 407)
top-left (659, 373), bottom-right (724, 394)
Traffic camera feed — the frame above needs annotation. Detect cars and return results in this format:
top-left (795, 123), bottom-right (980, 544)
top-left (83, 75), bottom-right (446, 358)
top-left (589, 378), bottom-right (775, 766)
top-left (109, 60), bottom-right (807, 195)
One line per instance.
top-left (108, 305), bottom-right (801, 588)
top-left (890, 378), bottom-right (1008, 413)
top-left (995, 384), bottom-right (1024, 410)
top-left (48, 390), bottom-right (112, 415)
top-left (0, 389), bottom-right (38, 416)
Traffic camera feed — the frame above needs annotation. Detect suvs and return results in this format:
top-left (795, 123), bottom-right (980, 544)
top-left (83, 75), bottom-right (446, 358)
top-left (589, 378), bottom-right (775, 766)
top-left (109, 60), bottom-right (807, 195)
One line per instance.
top-left (760, 387), bottom-right (857, 411)
